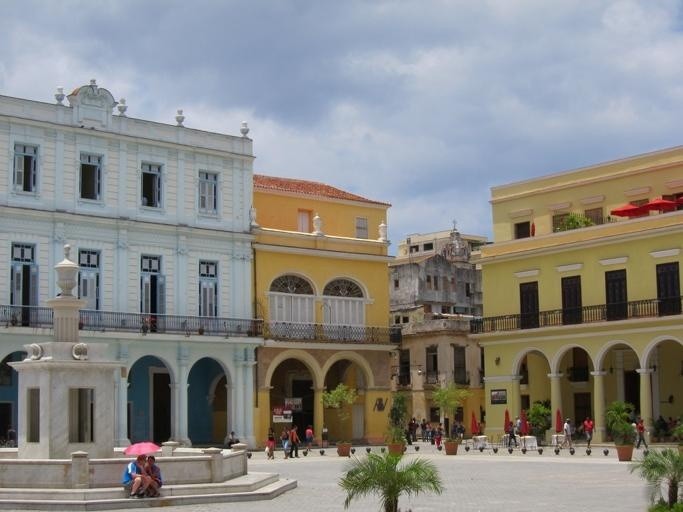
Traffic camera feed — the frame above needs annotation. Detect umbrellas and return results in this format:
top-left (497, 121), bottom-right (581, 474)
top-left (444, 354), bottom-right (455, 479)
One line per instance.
top-left (471, 411), bottom-right (480, 436)
top-left (611, 197), bottom-right (683, 217)
top-left (123, 442), bottom-right (160, 455)
top-left (519, 409), bottom-right (527, 433)
top-left (556, 408), bottom-right (561, 434)
top-left (505, 408), bottom-right (510, 433)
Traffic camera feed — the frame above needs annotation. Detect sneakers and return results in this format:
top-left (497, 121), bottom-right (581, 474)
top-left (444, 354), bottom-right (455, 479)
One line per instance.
top-left (129, 491), bottom-right (161, 499)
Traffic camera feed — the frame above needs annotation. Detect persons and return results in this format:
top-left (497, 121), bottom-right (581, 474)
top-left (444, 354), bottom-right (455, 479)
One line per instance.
top-left (306, 425), bottom-right (315, 451)
top-left (583, 416), bottom-right (594, 448)
top-left (122, 454), bottom-right (162, 498)
top-left (266, 428), bottom-right (276, 459)
top-left (632, 416), bottom-right (649, 449)
top-left (514, 416), bottom-right (521, 435)
top-left (404, 419), bottom-right (465, 448)
top-left (224, 431), bottom-right (252, 458)
top-left (508, 421), bottom-right (517, 447)
top-left (561, 417), bottom-right (571, 446)
top-left (8, 425), bottom-right (16, 448)
top-left (280, 425), bottom-right (300, 459)
top-left (657, 415), bottom-right (681, 442)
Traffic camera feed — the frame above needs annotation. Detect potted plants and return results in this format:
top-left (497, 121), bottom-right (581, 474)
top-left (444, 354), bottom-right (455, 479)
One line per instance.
top-left (524, 402), bottom-right (552, 446)
top-left (601, 400), bottom-right (640, 461)
top-left (321, 380), bottom-right (474, 458)
